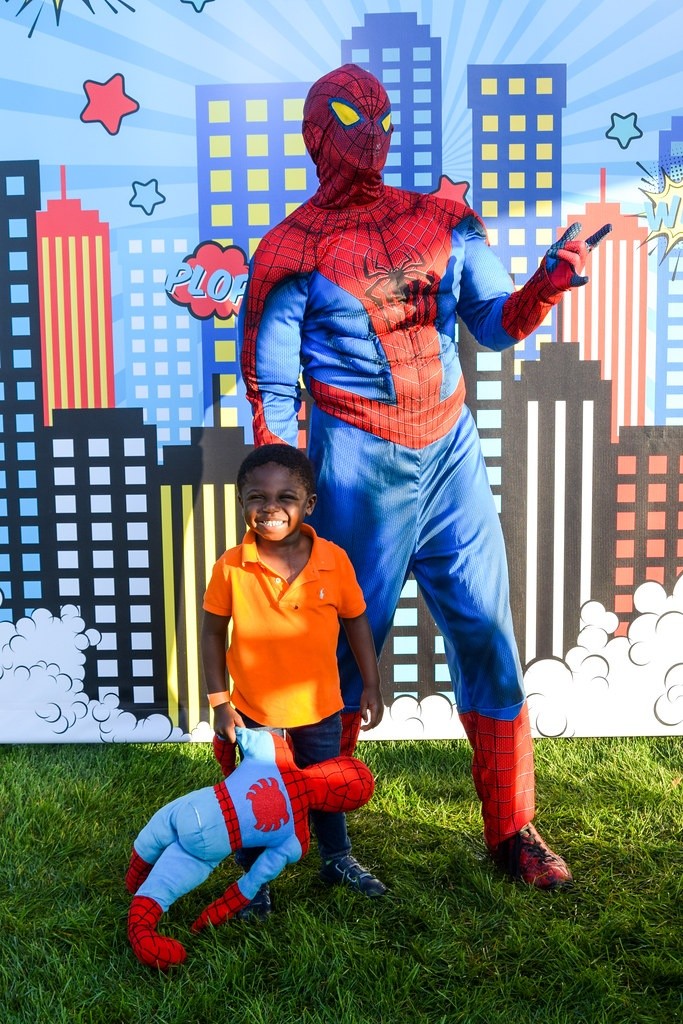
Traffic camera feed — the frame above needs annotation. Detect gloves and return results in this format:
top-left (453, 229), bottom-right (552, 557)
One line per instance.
top-left (545, 221), bottom-right (611, 290)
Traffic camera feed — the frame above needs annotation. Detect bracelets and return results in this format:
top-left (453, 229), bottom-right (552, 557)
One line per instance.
top-left (207, 689), bottom-right (231, 708)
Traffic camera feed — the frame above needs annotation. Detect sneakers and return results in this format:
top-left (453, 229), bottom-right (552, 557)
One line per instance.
top-left (237, 884), bottom-right (272, 923)
top-left (320, 856), bottom-right (386, 898)
top-left (493, 822), bottom-right (574, 891)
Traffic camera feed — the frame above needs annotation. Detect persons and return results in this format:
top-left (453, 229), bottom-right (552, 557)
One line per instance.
top-left (200, 441), bottom-right (386, 918)
top-left (236, 64), bottom-right (611, 889)
top-left (125, 726), bottom-right (374, 968)
